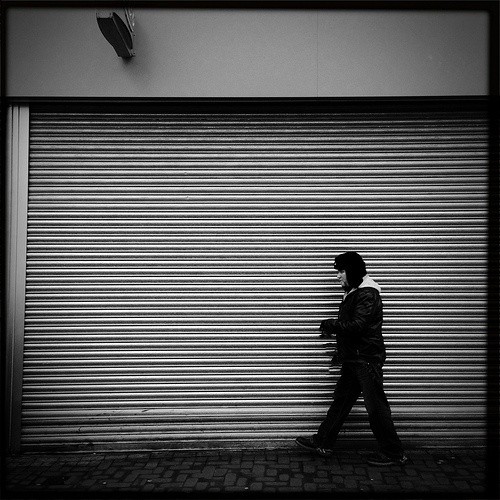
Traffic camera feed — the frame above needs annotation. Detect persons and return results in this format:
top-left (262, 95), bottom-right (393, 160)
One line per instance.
top-left (295, 252), bottom-right (409, 466)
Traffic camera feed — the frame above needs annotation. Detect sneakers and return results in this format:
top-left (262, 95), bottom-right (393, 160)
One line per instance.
top-left (369, 452), bottom-right (405, 465)
top-left (296, 436), bottom-right (332, 455)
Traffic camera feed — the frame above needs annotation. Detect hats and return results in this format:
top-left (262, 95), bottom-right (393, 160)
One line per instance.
top-left (334, 251), bottom-right (365, 269)
